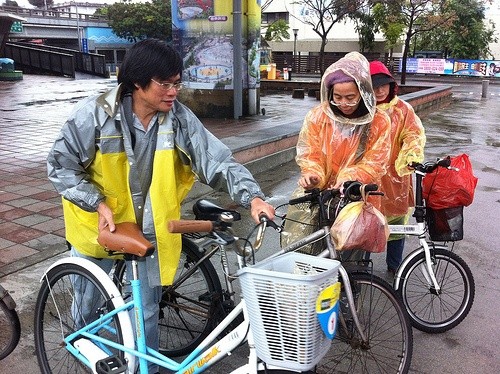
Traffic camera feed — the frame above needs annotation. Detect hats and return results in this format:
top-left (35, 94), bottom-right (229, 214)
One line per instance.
top-left (372, 74), bottom-right (396, 89)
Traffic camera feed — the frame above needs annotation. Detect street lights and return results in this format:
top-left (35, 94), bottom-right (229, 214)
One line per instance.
top-left (412, 23), bottom-right (421, 58)
top-left (293, 28), bottom-right (300, 73)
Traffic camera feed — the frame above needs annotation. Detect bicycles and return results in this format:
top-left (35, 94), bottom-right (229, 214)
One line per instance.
top-left (0, 285), bottom-right (22, 360)
top-left (31, 210), bottom-right (342, 374)
top-left (109, 180), bottom-right (414, 374)
top-left (268, 153), bottom-right (476, 334)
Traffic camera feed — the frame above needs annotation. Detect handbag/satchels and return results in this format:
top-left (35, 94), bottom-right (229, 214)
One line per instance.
top-left (330, 184), bottom-right (391, 253)
top-left (422, 154), bottom-right (478, 209)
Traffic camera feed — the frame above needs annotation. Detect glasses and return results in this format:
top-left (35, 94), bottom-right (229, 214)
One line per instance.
top-left (151, 79), bottom-right (186, 91)
top-left (328, 89), bottom-right (361, 106)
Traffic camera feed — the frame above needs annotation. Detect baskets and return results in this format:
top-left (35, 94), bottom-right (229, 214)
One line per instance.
top-left (236, 251), bottom-right (342, 372)
top-left (427, 205), bottom-right (464, 241)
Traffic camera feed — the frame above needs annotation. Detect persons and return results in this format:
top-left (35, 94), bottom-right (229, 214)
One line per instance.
top-left (47, 39), bottom-right (277, 374)
top-left (295, 52), bottom-right (391, 340)
top-left (366, 61), bottom-right (427, 274)
